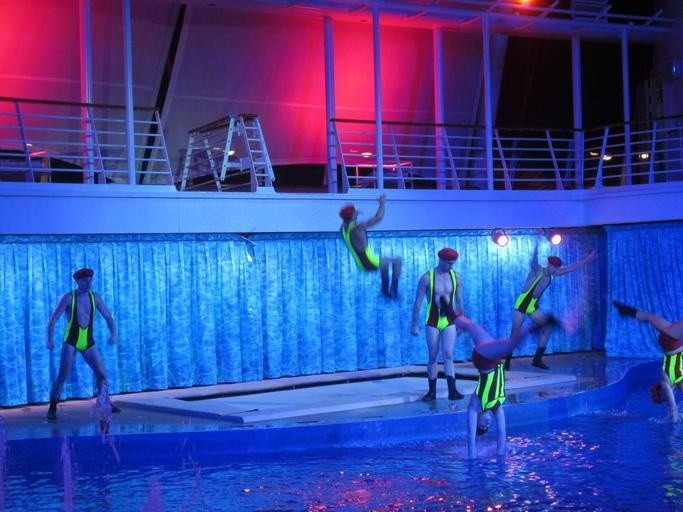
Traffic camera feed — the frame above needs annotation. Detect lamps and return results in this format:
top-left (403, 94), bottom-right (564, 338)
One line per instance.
top-left (490, 227), bottom-right (510, 248)
top-left (543, 226), bottom-right (565, 246)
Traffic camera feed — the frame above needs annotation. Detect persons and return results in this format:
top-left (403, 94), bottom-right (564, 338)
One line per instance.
top-left (501, 236), bottom-right (600, 371)
top-left (654, 424), bottom-right (682, 510)
top-left (610, 295), bottom-right (681, 424)
top-left (446, 309), bottom-right (567, 462)
top-left (408, 247), bottom-right (466, 405)
top-left (334, 189), bottom-right (404, 302)
top-left (41, 266), bottom-right (122, 423)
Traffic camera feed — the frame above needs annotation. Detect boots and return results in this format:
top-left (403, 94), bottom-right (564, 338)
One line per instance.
top-left (504, 352), bottom-right (513, 372)
top-left (446, 375), bottom-right (464, 401)
top-left (96, 387), bottom-right (122, 414)
top-left (422, 377), bottom-right (437, 402)
top-left (611, 300), bottom-right (639, 321)
top-left (46, 398), bottom-right (60, 423)
top-left (527, 311), bottom-right (564, 335)
top-left (390, 276), bottom-right (403, 300)
top-left (381, 277), bottom-right (391, 298)
top-left (439, 294), bottom-right (463, 323)
top-left (531, 346), bottom-right (551, 370)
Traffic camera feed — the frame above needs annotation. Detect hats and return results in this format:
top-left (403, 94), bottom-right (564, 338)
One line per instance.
top-left (547, 255), bottom-right (563, 268)
top-left (338, 204), bottom-right (356, 220)
top-left (649, 382), bottom-right (663, 405)
top-left (72, 268), bottom-right (95, 281)
top-left (475, 426), bottom-right (490, 436)
top-left (437, 247), bottom-right (459, 261)
